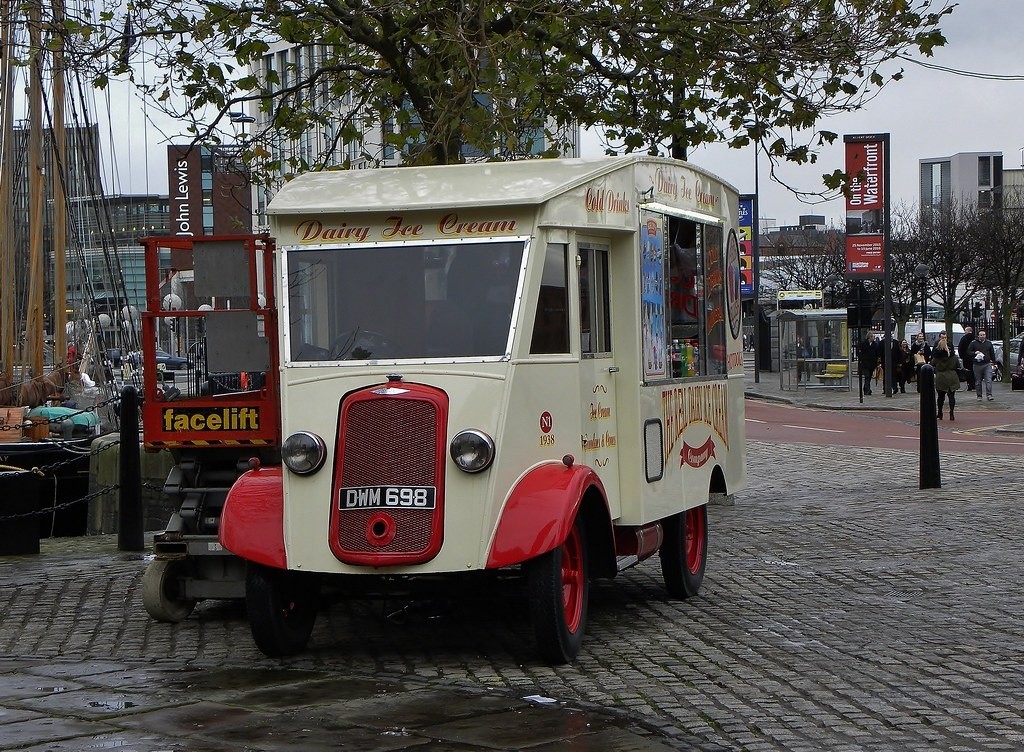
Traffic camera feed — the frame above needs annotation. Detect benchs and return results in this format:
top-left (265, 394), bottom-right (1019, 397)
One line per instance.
top-left (815, 364), bottom-right (849, 390)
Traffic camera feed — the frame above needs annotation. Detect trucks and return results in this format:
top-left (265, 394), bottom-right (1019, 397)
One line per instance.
top-left (132, 158), bottom-right (750, 666)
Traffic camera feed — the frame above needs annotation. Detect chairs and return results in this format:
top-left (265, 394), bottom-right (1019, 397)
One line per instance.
top-left (334, 250), bottom-right (550, 357)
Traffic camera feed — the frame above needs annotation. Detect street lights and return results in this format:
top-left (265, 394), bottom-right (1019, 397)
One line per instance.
top-left (915, 264), bottom-right (931, 343)
top-left (61, 292), bottom-right (266, 346)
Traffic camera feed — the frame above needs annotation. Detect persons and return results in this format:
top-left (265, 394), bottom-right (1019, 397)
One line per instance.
top-left (1017, 336), bottom-right (1024, 375)
top-left (796, 335), bottom-right (811, 382)
top-left (911, 332), bottom-right (931, 392)
top-left (958, 326), bottom-right (980, 391)
top-left (897, 339), bottom-right (914, 393)
top-left (747, 331), bottom-right (756, 352)
top-left (743, 333), bottom-right (748, 351)
top-left (932, 330), bottom-right (955, 359)
top-left (878, 331), bottom-right (901, 395)
top-left (966, 330), bottom-right (999, 401)
top-left (859, 331), bottom-right (877, 395)
top-left (931, 339), bottom-right (960, 421)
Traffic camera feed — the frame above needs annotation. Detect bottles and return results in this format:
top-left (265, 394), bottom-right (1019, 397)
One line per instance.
top-left (667, 338), bottom-right (700, 377)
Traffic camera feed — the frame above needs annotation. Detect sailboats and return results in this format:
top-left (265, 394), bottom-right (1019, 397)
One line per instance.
top-left (1, 1), bottom-right (179, 539)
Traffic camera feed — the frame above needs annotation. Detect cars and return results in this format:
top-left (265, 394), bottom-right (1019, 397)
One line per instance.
top-left (993, 337), bottom-right (1023, 385)
top-left (122, 347), bottom-right (193, 371)
top-left (870, 331), bottom-right (894, 343)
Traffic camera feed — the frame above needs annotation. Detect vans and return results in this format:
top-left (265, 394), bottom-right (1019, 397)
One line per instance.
top-left (894, 322), bottom-right (971, 381)
